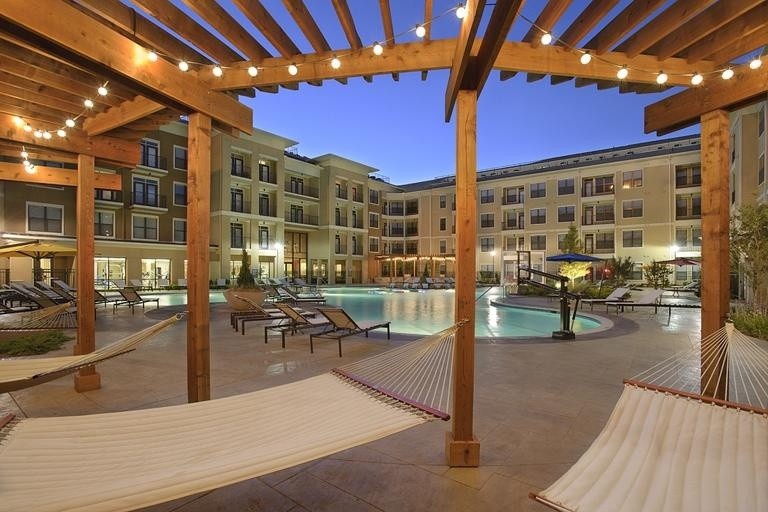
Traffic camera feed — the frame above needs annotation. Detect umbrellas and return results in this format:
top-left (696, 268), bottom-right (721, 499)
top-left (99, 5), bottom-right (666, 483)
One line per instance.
top-left (0, 239), bottom-right (102, 281)
top-left (545, 251), bottom-right (608, 265)
top-left (650, 257), bottom-right (700, 267)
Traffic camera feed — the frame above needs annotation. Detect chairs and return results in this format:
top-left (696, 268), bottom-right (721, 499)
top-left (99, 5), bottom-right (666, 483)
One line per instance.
top-left (230, 285), bottom-right (391, 357)
top-left (580, 282), bottom-right (699, 316)
top-left (0, 278), bottom-right (226, 322)
